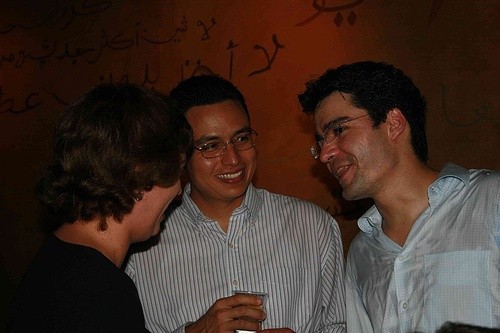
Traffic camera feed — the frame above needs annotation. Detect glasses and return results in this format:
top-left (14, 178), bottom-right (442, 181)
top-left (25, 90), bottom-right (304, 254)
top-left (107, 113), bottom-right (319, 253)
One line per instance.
top-left (192, 126), bottom-right (259, 160)
top-left (309, 113), bottom-right (369, 160)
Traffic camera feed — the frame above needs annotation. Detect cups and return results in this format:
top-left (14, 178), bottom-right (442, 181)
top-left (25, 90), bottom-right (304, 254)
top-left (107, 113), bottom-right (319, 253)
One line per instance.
top-left (233, 290), bottom-right (267, 333)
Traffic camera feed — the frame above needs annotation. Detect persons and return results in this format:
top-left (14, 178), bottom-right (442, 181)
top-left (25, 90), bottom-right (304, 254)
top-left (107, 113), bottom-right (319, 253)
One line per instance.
top-left (124, 74), bottom-right (347, 333)
top-left (298, 60), bottom-right (500, 333)
top-left (0, 82), bottom-right (194, 333)
